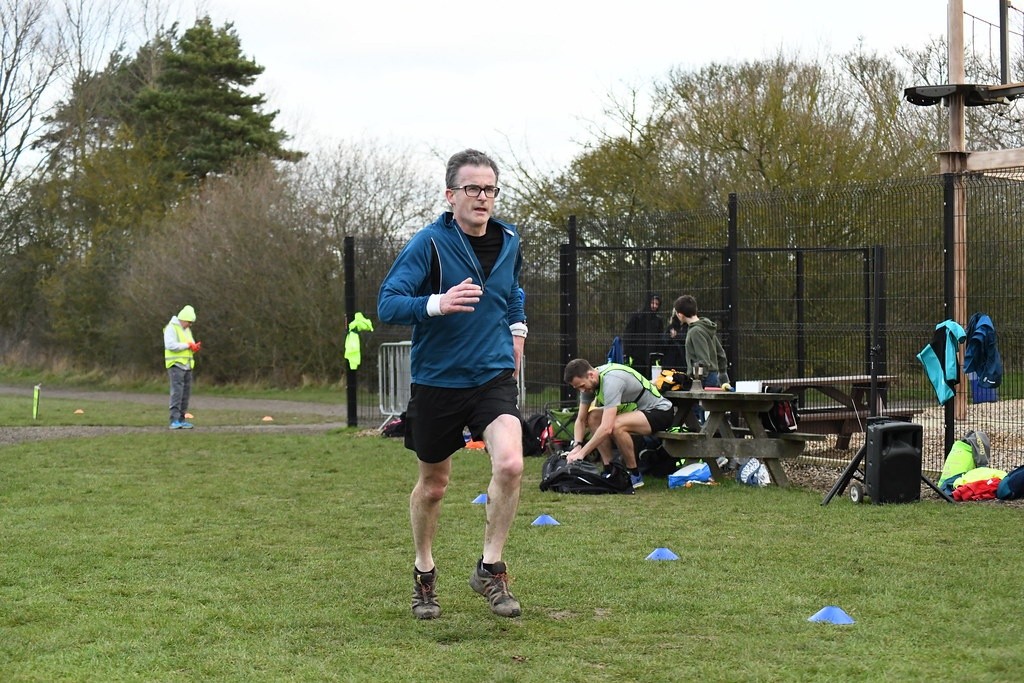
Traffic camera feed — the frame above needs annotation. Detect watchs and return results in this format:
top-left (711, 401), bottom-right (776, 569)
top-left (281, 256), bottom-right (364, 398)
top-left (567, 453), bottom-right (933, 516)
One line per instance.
top-left (573, 441), bottom-right (584, 448)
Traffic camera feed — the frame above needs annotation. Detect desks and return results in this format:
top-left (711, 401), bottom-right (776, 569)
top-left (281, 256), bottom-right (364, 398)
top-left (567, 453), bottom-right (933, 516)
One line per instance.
top-left (660, 392), bottom-right (793, 487)
top-left (758, 374), bottom-right (897, 450)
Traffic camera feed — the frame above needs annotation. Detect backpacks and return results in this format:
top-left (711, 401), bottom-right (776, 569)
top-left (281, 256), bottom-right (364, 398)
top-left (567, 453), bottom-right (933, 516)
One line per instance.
top-left (521, 414), bottom-right (554, 457)
top-left (537, 466), bottom-right (631, 494)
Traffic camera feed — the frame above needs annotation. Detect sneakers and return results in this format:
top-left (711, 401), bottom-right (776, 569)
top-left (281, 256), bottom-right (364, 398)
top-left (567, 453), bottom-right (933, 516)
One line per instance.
top-left (169, 420), bottom-right (182, 429)
top-left (599, 471), bottom-right (611, 479)
top-left (411, 568), bottom-right (440, 620)
top-left (178, 422), bottom-right (193, 428)
top-left (627, 470), bottom-right (645, 489)
top-left (470, 555), bottom-right (521, 617)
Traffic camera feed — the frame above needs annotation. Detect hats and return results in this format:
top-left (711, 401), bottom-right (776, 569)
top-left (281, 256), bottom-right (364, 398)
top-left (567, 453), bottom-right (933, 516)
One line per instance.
top-left (178, 305), bottom-right (196, 322)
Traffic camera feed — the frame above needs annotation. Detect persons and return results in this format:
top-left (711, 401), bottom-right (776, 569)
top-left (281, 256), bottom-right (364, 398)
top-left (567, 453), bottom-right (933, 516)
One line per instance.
top-left (674, 295), bottom-right (730, 426)
top-left (163, 305), bottom-right (201, 430)
top-left (377, 148), bottom-right (528, 619)
top-left (622, 291), bottom-right (665, 381)
top-left (662, 306), bottom-right (689, 374)
top-left (564, 358), bottom-right (675, 489)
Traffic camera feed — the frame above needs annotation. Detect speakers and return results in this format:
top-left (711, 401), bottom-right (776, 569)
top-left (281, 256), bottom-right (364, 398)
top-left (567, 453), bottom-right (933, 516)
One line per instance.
top-left (867, 421), bottom-right (923, 505)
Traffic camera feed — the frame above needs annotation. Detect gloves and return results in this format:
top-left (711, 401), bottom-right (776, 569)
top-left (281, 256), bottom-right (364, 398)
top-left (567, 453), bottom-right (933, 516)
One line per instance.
top-left (189, 341), bottom-right (201, 352)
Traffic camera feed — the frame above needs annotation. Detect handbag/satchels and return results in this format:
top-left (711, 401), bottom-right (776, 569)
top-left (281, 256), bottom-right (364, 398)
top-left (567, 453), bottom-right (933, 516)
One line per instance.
top-left (937, 439), bottom-right (976, 497)
top-left (541, 451), bottom-right (602, 482)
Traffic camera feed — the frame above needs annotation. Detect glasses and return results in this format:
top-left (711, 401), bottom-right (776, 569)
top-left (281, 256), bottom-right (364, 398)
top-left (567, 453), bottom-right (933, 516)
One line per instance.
top-left (450, 184), bottom-right (500, 198)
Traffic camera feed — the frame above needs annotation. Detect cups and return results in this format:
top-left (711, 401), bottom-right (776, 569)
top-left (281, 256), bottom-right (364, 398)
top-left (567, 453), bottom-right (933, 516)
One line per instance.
top-left (651, 365), bottom-right (662, 383)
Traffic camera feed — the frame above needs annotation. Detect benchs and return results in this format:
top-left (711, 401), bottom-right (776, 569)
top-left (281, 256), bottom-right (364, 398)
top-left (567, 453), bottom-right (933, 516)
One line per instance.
top-left (624, 432), bottom-right (706, 479)
top-left (789, 403), bottom-right (924, 450)
top-left (701, 426), bottom-right (826, 489)
